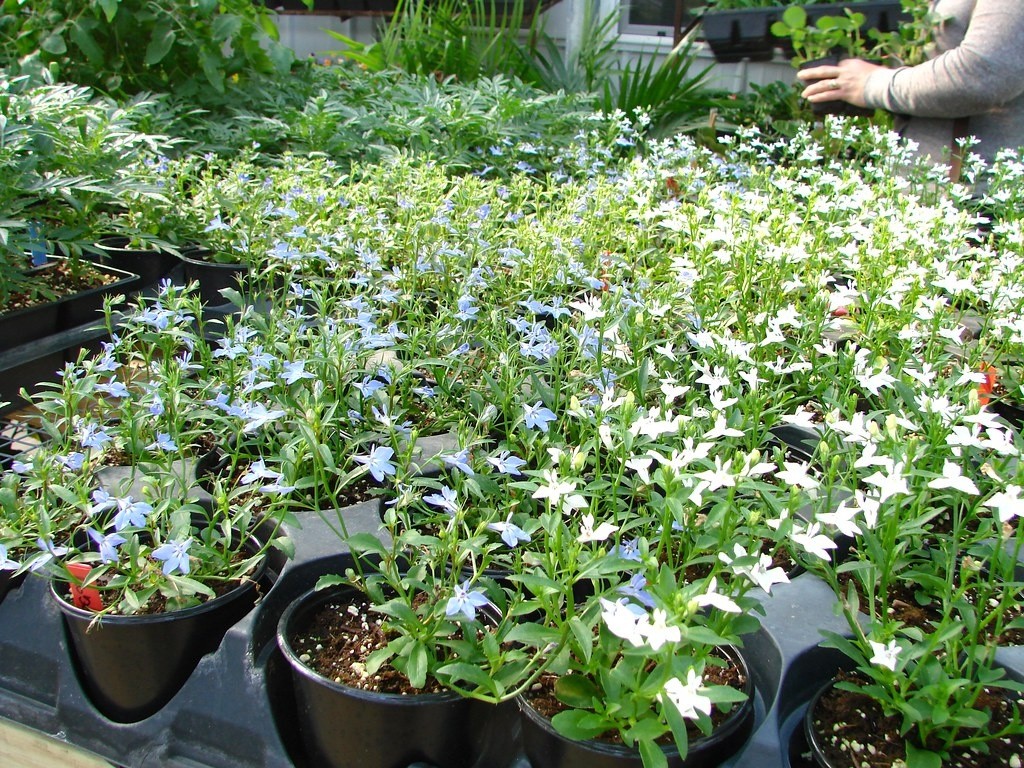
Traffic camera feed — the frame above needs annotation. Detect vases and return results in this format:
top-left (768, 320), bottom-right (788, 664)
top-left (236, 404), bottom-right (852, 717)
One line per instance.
top-left (92, 237), bottom-right (181, 286)
top-left (45, 523), bottom-right (265, 725)
top-left (515, 605), bottom-right (754, 768)
top-left (572, 424), bottom-right (852, 612)
top-left (277, 574), bottom-right (519, 768)
top-left (0, 249), bottom-right (140, 369)
top-left (181, 251), bottom-right (256, 306)
top-left (802, 659), bottom-right (1024, 768)
top-left (0, 311), bottom-right (139, 418)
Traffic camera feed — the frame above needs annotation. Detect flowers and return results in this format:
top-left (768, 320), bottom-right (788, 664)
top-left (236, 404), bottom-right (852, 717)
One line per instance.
top-left (0, 115), bottom-right (1024, 768)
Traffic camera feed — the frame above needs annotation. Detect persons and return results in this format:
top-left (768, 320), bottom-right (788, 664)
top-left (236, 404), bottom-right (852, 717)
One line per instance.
top-left (797, 0), bottom-right (1024, 248)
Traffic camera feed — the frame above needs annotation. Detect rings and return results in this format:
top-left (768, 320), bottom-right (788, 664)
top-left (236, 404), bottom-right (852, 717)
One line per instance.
top-left (830, 79), bottom-right (836, 89)
